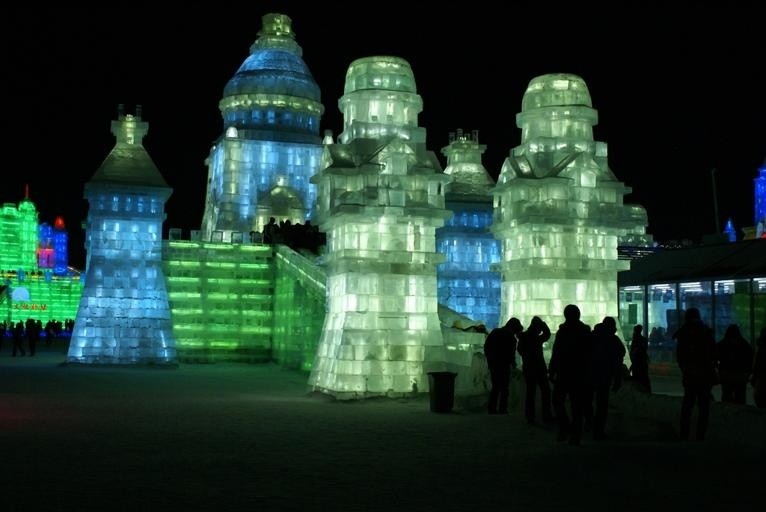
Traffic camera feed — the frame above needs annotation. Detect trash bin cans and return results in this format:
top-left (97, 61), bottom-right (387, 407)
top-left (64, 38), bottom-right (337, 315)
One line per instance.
top-left (427, 371), bottom-right (458, 412)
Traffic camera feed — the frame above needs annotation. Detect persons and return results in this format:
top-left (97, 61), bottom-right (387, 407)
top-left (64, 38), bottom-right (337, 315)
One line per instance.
top-left (716, 322), bottom-right (753, 405)
top-left (250, 215), bottom-right (327, 260)
top-left (515, 315), bottom-right (551, 428)
top-left (583, 316), bottom-right (625, 442)
top-left (631, 343), bottom-right (653, 394)
top-left (546, 303), bottom-right (590, 441)
top-left (675, 306), bottom-right (718, 442)
top-left (0, 314), bottom-right (75, 359)
top-left (482, 316), bottom-right (523, 415)
top-left (626, 324), bottom-right (642, 373)
top-left (750, 325), bottom-right (765, 409)
